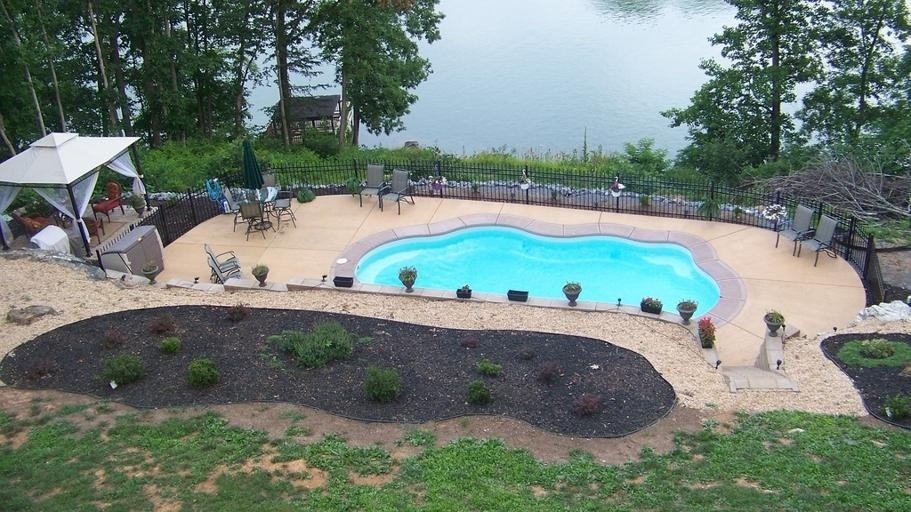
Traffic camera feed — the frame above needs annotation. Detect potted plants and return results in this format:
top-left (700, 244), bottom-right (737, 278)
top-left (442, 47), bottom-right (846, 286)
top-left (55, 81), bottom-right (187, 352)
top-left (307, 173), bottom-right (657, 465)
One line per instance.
top-left (130, 194), bottom-right (146, 218)
top-left (251, 264), bottom-right (268, 287)
top-left (695, 316), bottom-right (715, 347)
top-left (562, 281), bottom-right (581, 306)
top-left (763, 312), bottom-right (783, 337)
top-left (641, 295), bottom-right (697, 325)
top-left (398, 265), bottom-right (417, 293)
top-left (140, 259), bottom-right (160, 285)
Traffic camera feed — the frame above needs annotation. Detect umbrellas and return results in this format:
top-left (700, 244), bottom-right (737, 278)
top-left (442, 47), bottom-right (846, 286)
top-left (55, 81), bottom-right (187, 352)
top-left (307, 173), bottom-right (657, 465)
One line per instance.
top-left (243, 138), bottom-right (264, 190)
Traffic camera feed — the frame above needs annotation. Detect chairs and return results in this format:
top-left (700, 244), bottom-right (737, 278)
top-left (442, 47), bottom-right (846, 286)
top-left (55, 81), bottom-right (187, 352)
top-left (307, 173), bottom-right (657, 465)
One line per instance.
top-left (355, 164), bottom-right (414, 215)
top-left (202, 243), bottom-right (241, 284)
top-left (220, 171), bottom-right (296, 242)
top-left (10, 212), bottom-right (55, 239)
top-left (775, 204), bottom-right (841, 267)
top-left (89, 181), bottom-right (124, 224)
